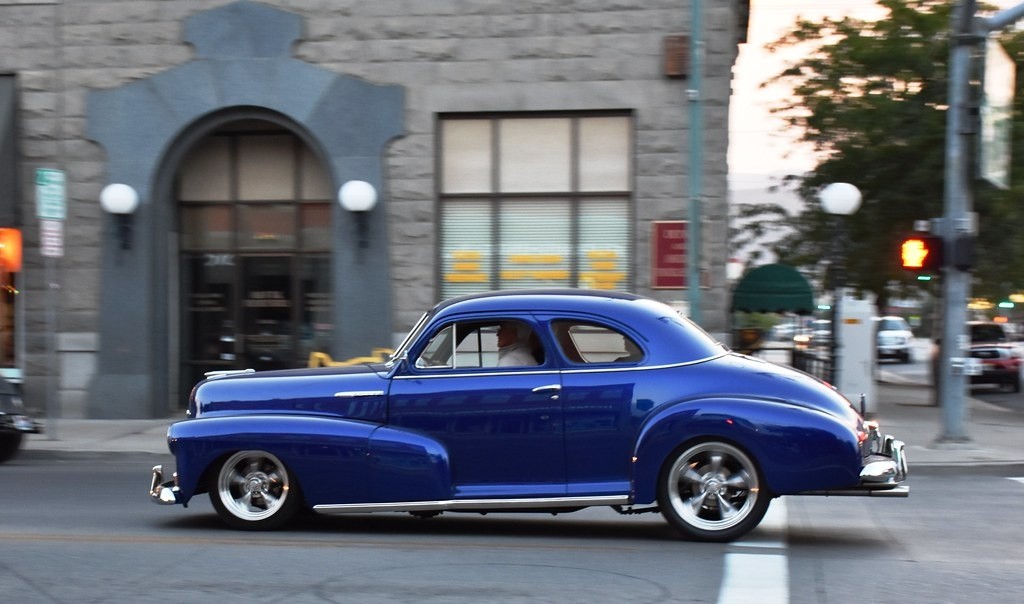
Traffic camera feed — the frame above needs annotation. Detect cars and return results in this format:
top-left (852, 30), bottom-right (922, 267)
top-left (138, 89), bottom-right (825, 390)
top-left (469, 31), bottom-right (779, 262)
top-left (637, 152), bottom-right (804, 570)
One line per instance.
top-left (150, 289), bottom-right (913, 545)
top-left (932, 320), bottom-right (1023, 395)
top-left (772, 320), bottom-right (832, 366)
top-left (0, 366), bottom-right (45, 461)
top-left (873, 313), bottom-right (915, 365)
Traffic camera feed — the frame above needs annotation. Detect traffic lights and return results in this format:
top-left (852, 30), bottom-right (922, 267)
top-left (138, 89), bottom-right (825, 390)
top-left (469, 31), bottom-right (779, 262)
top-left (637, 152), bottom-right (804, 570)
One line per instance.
top-left (900, 234), bottom-right (942, 270)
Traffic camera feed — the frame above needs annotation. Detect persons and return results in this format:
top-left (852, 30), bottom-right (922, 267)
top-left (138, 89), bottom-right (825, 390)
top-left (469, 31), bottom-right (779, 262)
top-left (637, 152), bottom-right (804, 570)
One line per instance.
top-left (496, 323), bottom-right (538, 367)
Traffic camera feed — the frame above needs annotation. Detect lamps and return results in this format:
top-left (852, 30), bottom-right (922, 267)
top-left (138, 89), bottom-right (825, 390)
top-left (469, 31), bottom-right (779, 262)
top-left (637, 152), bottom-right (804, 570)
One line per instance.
top-left (337, 178), bottom-right (378, 255)
top-left (96, 183), bottom-right (140, 254)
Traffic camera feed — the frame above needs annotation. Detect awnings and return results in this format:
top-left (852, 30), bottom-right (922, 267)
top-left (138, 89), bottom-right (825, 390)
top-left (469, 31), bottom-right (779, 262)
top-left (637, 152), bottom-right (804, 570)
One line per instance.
top-left (730, 264), bottom-right (813, 315)
